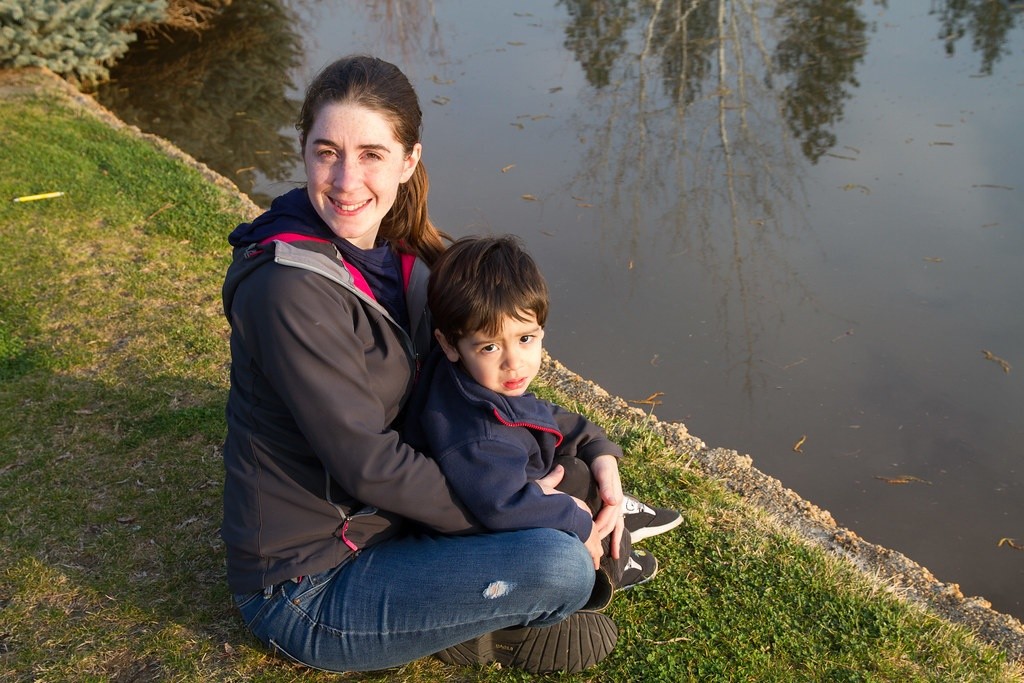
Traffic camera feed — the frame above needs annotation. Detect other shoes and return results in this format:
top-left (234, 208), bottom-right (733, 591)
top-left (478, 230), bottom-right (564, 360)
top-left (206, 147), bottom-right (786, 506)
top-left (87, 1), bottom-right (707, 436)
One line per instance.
top-left (622, 505), bottom-right (684, 543)
top-left (611, 548), bottom-right (658, 594)
top-left (436, 611), bottom-right (619, 671)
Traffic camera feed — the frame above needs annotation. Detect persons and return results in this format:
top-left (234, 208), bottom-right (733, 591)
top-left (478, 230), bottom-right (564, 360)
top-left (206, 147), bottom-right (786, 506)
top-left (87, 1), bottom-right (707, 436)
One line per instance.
top-left (217, 55), bottom-right (620, 675)
top-left (414, 231), bottom-right (684, 598)
